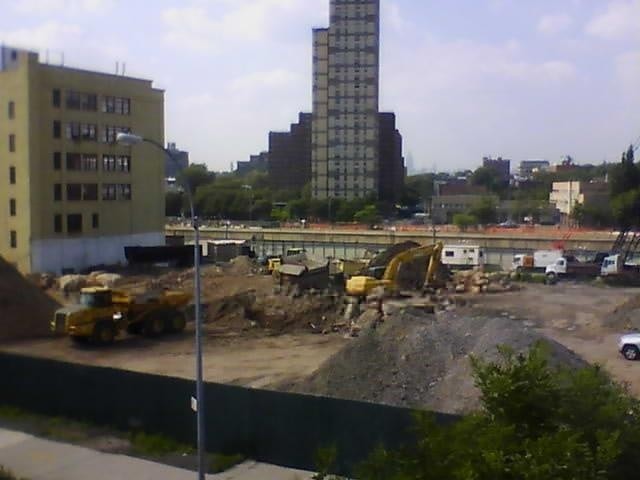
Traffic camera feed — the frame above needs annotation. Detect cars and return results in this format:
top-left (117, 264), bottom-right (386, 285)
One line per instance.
top-left (619, 334), bottom-right (640, 360)
top-left (496, 220), bottom-right (520, 228)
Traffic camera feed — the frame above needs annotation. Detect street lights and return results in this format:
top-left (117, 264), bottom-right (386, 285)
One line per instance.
top-left (116, 131), bottom-right (206, 479)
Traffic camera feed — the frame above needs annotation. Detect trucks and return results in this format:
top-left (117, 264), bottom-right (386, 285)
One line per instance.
top-left (511, 248), bottom-right (640, 285)
top-left (440, 245), bottom-right (483, 271)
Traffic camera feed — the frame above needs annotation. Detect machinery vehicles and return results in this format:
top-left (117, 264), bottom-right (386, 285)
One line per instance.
top-left (251, 254), bottom-right (282, 274)
top-left (52, 284), bottom-right (192, 348)
top-left (343, 244), bottom-right (444, 313)
